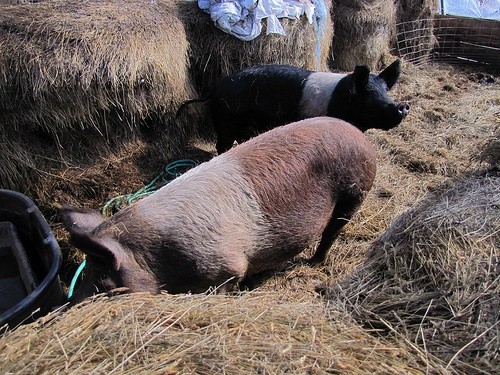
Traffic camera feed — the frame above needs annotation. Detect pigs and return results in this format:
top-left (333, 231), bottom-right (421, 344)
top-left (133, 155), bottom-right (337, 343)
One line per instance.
top-left (61, 117), bottom-right (378, 312)
top-left (185, 59), bottom-right (410, 158)
